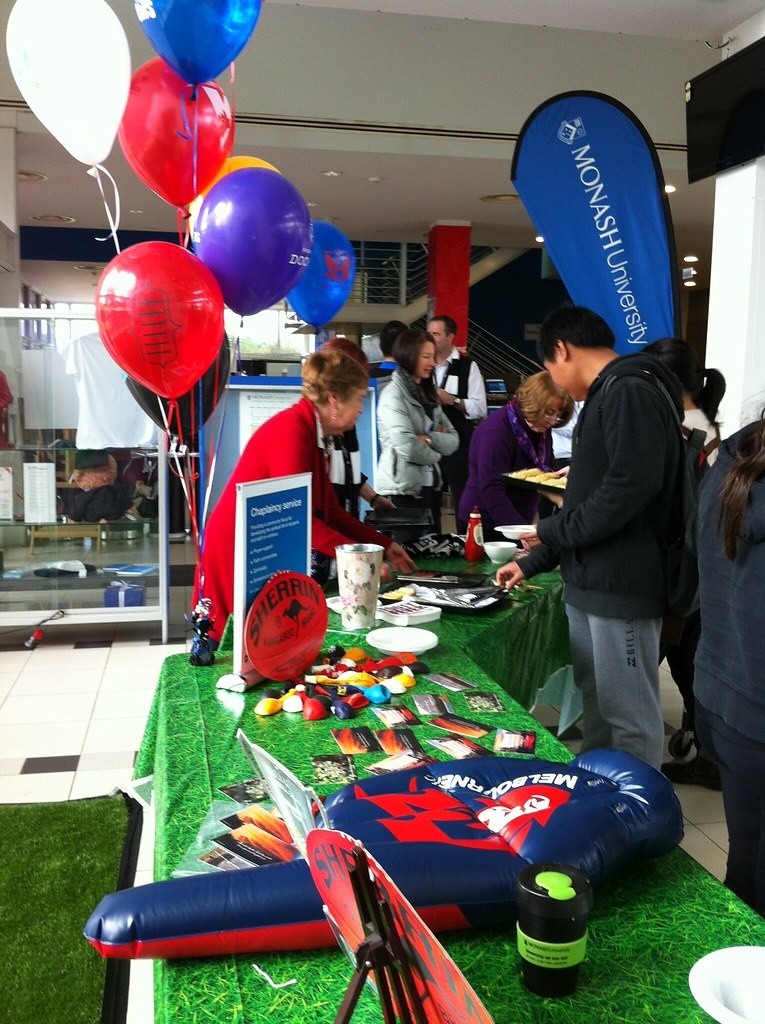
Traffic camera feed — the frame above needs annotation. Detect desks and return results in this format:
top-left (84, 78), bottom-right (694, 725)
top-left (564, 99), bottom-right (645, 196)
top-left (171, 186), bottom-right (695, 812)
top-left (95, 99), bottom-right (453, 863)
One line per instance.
top-left (217, 555), bottom-right (584, 739)
top-left (132, 640), bottom-right (765, 1024)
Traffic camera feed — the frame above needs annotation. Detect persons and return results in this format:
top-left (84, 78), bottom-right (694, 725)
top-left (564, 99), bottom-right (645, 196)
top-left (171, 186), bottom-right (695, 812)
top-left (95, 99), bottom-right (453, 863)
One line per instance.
top-left (459, 371), bottom-right (575, 549)
top-left (311, 338), bottom-right (397, 581)
top-left (497, 306), bottom-right (727, 772)
top-left (192, 348), bottom-right (419, 651)
top-left (379, 320), bottom-right (409, 370)
top-left (428, 315), bottom-right (487, 534)
top-left (376, 328), bottom-right (459, 544)
top-left (691, 418), bottom-right (765, 917)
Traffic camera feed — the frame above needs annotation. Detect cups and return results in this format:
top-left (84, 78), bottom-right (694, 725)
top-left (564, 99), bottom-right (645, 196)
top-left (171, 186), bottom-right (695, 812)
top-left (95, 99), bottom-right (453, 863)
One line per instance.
top-left (335, 543), bottom-right (385, 630)
top-left (516, 861), bottom-right (593, 998)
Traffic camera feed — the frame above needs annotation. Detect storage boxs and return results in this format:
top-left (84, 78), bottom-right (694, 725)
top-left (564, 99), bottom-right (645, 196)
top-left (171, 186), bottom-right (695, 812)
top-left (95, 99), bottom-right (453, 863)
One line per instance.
top-left (104, 580), bottom-right (147, 606)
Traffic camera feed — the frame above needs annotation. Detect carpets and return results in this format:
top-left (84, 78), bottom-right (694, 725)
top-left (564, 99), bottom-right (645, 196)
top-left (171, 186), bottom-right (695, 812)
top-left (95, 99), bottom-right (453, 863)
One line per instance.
top-left (0, 787), bottom-right (144, 1024)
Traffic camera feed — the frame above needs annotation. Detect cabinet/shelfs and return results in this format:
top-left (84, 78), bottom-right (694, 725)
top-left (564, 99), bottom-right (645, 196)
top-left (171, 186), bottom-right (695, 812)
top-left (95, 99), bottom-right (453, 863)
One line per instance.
top-left (0, 306), bottom-right (171, 644)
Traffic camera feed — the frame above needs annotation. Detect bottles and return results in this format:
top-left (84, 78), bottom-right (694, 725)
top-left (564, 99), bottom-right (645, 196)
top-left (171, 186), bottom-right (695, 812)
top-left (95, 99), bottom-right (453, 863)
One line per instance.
top-left (464, 504), bottom-right (484, 561)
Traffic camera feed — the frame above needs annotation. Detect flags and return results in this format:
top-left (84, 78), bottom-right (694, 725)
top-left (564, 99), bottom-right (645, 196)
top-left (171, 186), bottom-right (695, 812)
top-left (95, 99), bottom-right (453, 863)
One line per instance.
top-left (512, 98), bottom-right (675, 356)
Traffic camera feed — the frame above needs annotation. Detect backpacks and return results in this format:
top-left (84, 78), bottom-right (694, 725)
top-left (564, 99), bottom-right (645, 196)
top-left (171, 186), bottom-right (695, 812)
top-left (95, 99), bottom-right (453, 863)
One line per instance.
top-left (601, 368), bottom-right (707, 618)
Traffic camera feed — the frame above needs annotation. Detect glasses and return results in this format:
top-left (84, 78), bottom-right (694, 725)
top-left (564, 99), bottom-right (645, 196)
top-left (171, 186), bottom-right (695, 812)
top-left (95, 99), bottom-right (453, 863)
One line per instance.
top-left (543, 414), bottom-right (565, 425)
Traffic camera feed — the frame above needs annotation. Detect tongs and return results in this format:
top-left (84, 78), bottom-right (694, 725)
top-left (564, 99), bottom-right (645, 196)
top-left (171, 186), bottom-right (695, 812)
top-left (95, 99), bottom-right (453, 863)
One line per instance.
top-left (396, 575), bottom-right (462, 584)
top-left (446, 583), bottom-right (504, 605)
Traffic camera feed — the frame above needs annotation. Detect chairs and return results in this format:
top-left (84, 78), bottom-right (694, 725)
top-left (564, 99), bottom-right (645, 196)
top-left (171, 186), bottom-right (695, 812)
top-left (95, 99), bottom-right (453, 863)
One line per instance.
top-left (29, 430), bottom-right (111, 556)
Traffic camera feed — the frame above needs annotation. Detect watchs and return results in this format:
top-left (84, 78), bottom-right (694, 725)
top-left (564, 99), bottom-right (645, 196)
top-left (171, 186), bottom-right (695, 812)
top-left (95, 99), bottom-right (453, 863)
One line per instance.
top-left (453, 398), bottom-right (460, 406)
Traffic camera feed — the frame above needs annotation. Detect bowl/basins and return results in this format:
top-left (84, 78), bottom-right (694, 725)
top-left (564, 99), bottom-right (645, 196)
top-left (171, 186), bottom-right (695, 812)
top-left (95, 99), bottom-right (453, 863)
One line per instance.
top-left (366, 627), bottom-right (439, 655)
top-left (689, 945), bottom-right (765, 1024)
top-left (484, 541), bottom-right (518, 564)
top-left (496, 525), bottom-right (539, 540)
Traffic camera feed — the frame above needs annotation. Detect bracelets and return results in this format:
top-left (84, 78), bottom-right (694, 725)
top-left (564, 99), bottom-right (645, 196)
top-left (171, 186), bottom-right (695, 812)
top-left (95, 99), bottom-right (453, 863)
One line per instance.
top-left (426, 434), bottom-right (430, 445)
top-left (370, 494), bottom-right (380, 507)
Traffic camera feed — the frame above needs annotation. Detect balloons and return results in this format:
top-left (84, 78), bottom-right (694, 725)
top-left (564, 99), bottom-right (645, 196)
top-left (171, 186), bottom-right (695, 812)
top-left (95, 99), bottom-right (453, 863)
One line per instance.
top-left (285, 221), bottom-right (356, 329)
top-left (117, 57), bottom-right (234, 220)
top-left (130, 0), bottom-right (263, 101)
top-left (123, 331), bottom-right (231, 438)
top-left (190, 155), bottom-right (313, 318)
top-left (94, 241), bottom-right (224, 407)
top-left (6, 0), bottom-right (132, 166)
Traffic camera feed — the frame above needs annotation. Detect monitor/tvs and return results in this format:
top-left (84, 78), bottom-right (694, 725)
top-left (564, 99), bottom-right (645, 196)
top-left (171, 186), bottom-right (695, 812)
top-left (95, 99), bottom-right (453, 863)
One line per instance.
top-left (686, 36), bottom-right (765, 185)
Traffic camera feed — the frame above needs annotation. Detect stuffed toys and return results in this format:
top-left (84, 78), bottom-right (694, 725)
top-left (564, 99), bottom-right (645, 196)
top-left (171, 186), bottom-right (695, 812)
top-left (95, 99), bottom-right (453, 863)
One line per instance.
top-left (68, 449), bottom-right (138, 525)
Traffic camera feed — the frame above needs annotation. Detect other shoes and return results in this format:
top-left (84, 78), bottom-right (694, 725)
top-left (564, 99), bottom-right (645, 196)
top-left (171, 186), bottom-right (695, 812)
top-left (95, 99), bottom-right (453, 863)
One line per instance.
top-left (661, 759), bottom-right (722, 791)
